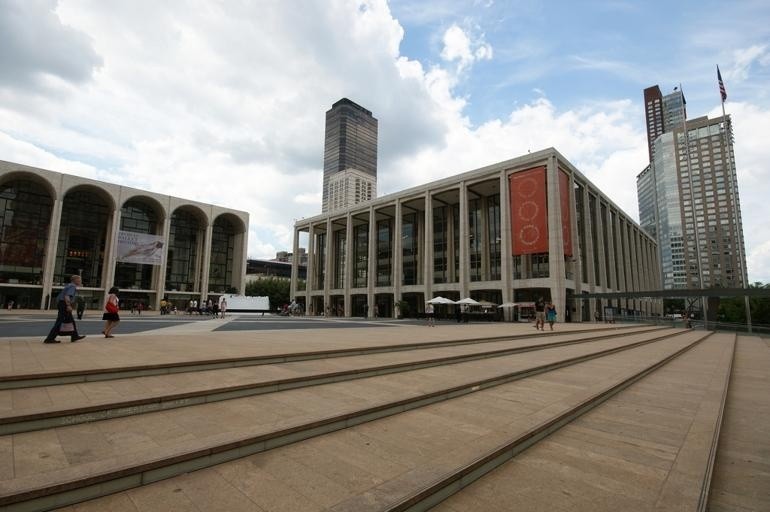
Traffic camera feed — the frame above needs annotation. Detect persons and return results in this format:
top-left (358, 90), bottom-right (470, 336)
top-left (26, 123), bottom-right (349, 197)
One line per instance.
top-left (534, 295), bottom-right (544, 331)
top-left (219, 298), bottom-right (227, 319)
top-left (456, 304), bottom-right (461, 323)
top-left (545, 299), bottom-right (557, 330)
top-left (425, 302), bottom-right (434, 328)
top-left (42, 274), bottom-right (86, 344)
top-left (130, 297), bottom-right (219, 319)
top-left (463, 303), bottom-right (468, 322)
top-left (76, 295), bottom-right (87, 320)
top-left (100, 285), bottom-right (121, 339)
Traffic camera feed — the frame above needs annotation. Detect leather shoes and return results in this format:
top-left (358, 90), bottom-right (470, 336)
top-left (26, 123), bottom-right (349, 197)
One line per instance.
top-left (44, 338), bottom-right (61, 343)
top-left (71, 336), bottom-right (84, 342)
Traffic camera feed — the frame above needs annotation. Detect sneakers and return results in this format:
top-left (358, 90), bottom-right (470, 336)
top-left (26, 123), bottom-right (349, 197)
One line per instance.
top-left (102, 331), bottom-right (105, 334)
top-left (105, 335), bottom-right (114, 338)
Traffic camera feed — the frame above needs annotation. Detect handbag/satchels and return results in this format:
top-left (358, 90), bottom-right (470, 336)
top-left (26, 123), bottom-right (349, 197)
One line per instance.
top-left (59, 322), bottom-right (75, 336)
top-left (106, 303), bottom-right (118, 314)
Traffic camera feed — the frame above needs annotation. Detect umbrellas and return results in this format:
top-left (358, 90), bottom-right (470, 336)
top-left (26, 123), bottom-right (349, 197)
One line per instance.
top-left (425, 295), bottom-right (454, 320)
top-left (454, 297), bottom-right (480, 307)
